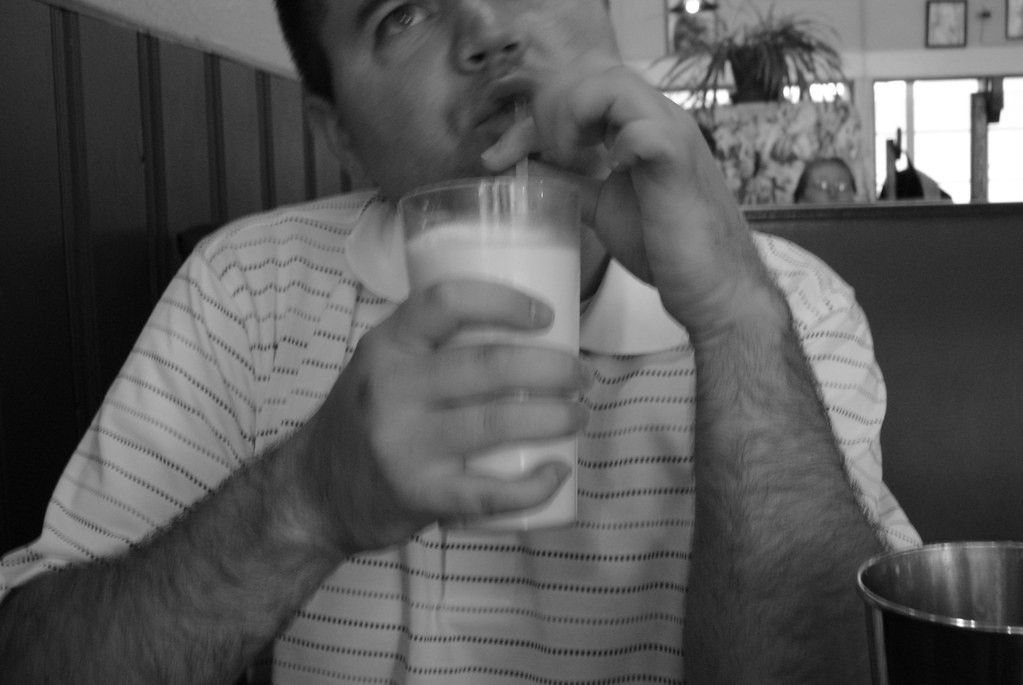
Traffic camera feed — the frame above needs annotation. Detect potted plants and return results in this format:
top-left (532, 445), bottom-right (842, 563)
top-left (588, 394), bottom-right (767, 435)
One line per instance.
top-left (653, 0), bottom-right (847, 122)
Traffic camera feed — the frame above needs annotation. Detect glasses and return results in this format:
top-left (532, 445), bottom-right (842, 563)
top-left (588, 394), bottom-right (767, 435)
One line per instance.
top-left (806, 178), bottom-right (853, 196)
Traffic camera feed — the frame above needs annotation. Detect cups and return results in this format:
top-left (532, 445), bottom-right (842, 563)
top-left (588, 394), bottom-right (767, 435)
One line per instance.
top-left (397, 179), bottom-right (583, 532)
top-left (854, 541), bottom-right (1023, 685)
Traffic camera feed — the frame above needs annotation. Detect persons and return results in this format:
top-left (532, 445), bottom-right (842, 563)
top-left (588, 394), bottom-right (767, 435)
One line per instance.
top-left (0, 0), bottom-right (923, 685)
top-left (793, 156), bottom-right (857, 204)
